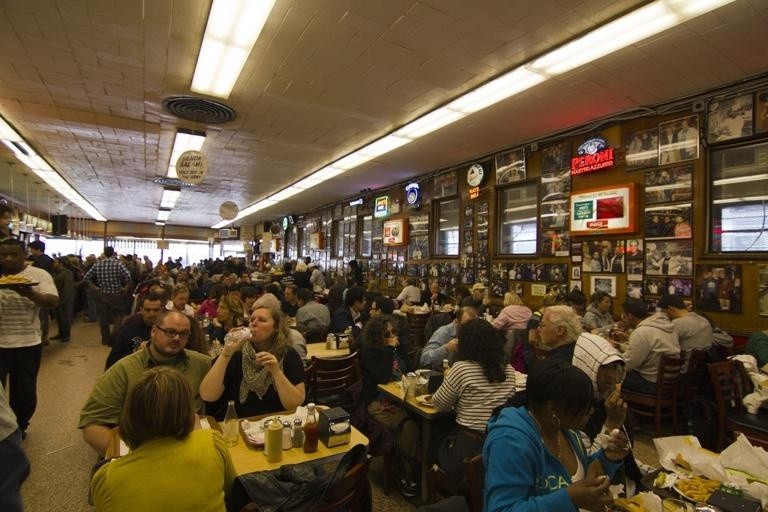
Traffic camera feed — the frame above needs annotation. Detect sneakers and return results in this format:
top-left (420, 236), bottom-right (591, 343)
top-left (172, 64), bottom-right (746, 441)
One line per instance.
top-left (400, 477), bottom-right (418, 497)
top-left (42, 336), bottom-right (70, 345)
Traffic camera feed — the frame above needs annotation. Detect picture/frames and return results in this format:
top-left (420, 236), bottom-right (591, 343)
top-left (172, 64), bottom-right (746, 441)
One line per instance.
top-left (281, 90), bottom-right (768, 316)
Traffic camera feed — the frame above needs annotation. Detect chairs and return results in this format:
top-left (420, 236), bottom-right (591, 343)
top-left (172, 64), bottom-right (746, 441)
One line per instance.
top-left (403, 309), bottom-right (462, 368)
top-left (245, 459), bottom-right (371, 511)
top-left (304, 350), bottom-right (360, 403)
top-left (621, 344), bottom-right (768, 452)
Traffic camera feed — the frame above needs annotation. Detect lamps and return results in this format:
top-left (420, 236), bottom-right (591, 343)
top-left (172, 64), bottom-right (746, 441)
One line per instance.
top-left (213, 166), bottom-right (345, 230)
top-left (156, 213), bottom-right (171, 227)
top-left (528, 0), bottom-right (733, 78)
top-left (328, 132), bottom-right (412, 171)
top-left (190, 1), bottom-right (275, 99)
top-left (160, 187), bottom-right (181, 210)
top-left (394, 64), bottom-right (547, 139)
top-left (167, 129), bottom-right (206, 178)
top-left (2, 118), bottom-right (108, 222)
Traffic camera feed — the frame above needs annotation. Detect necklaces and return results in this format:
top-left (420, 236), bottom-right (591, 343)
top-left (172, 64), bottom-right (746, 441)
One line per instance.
top-left (528, 409), bottom-right (563, 458)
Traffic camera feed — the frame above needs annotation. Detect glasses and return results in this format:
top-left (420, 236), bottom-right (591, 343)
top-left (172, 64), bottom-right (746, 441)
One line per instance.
top-left (153, 323), bottom-right (191, 340)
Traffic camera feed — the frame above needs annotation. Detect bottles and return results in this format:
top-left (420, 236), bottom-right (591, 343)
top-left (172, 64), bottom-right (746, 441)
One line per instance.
top-left (224, 327), bottom-right (253, 346)
top-left (325, 334), bottom-right (337, 349)
top-left (406, 372), bottom-right (417, 402)
top-left (442, 359), bottom-right (449, 376)
top-left (348, 326), bottom-right (353, 334)
top-left (222, 401), bottom-right (318, 463)
top-left (203, 313), bottom-right (212, 343)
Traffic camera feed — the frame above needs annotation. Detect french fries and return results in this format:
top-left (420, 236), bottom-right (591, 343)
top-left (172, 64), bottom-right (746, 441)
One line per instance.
top-left (677, 475), bottom-right (721, 502)
top-left (0, 275), bottom-right (32, 283)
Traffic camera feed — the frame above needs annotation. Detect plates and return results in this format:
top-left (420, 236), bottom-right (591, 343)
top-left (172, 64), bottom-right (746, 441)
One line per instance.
top-left (672, 473), bottom-right (702, 504)
top-left (0, 282), bottom-right (41, 287)
top-left (416, 393), bottom-right (433, 407)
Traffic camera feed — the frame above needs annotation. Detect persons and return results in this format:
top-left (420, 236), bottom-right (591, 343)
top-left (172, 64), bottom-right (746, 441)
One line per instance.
top-left (629, 132), bottom-right (653, 165)
top-left (0, 240), bottom-right (768, 511)
top-left (647, 214), bottom-right (690, 235)
top-left (661, 120), bottom-right (698, 163)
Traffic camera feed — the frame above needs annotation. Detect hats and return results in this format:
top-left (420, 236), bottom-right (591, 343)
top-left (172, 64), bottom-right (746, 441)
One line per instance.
top-left (602, 356), bottom-right (626, 369)
top-left (473, 283), bottom-right (488, 292)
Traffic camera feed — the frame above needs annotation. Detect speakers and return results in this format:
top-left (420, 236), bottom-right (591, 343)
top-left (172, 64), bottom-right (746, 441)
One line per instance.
top-left (50, 214), bottom-right (68, 236)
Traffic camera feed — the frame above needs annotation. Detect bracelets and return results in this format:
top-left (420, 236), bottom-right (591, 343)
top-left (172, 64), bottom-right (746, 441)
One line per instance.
top-left (221, 351), bottom-right (231, 356)
